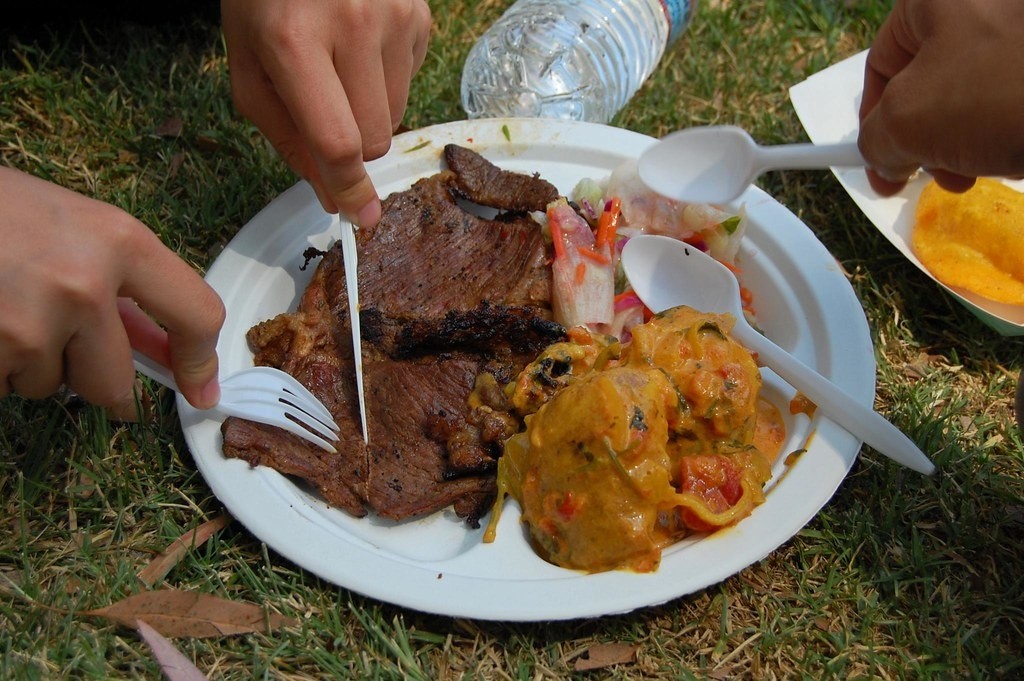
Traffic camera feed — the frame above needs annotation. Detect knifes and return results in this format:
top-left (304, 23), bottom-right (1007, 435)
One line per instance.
top-left (338, 209), bottom-right (368, 446)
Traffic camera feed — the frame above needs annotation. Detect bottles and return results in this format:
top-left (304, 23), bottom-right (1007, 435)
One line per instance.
top-left (459, 0), bottom-right (696, 125)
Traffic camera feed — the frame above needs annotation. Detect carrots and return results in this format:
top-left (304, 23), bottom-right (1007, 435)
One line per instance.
top-left (546, 197), bottom-right (757, 322)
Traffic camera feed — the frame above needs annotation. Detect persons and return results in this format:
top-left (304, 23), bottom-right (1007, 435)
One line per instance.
top-left (858, 0), bottom-right (1024, 199)
top-left (0, 0), bottom-right (433, 419)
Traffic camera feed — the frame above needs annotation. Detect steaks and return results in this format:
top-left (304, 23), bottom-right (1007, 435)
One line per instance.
top-left (219, 143), bottom-right (559, 529)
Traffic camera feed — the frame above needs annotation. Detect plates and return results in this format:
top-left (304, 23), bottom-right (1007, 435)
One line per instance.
top-left (174, 116), bottom-right (877, 625)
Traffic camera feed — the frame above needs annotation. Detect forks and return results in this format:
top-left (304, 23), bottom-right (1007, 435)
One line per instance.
top-left (130, 347), bottom-right (340, 455)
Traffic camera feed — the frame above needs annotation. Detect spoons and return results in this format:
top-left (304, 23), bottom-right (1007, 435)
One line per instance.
top-left (638, 124), bottom-right (872, 206)
top-left (620, 234), bottom-right (936, 476)
